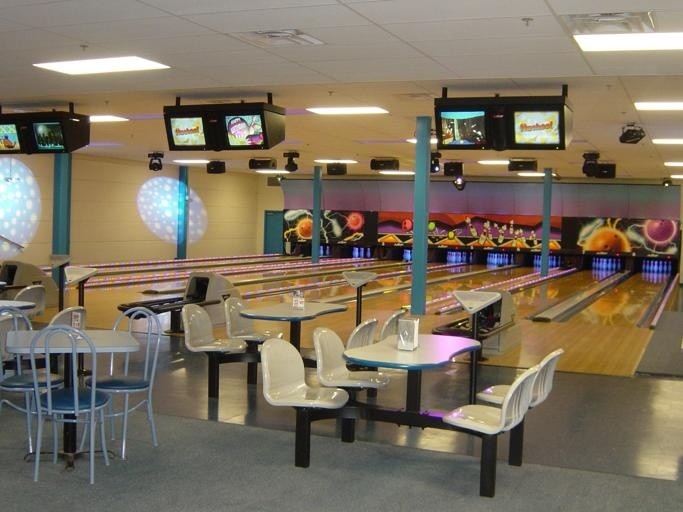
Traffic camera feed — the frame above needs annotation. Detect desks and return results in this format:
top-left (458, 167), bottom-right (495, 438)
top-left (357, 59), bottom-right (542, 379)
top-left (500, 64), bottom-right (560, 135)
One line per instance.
top-left (239, 300), bottom-right (348, 354)
top-left (5, 330), bottom-right (141, 470)
top-left (0, 298), bottom-right (37, 311)
top-left (345, 334), bottom-right (482, 411)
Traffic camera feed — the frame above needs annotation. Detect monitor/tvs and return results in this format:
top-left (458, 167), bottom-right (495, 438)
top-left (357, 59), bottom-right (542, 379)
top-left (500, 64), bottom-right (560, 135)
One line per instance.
top-left (161, 109), bottom-right (211, 150)
top-left (434, 105), bottom-right (490, 150)
top-left (29, 117), bottom-right (90, 153)
top-left (0, 117), bottom-right (24, 154)
top-left (507, 103), bottom-right (575, 150)
top-left (221, 110), bottom-right (285, 149)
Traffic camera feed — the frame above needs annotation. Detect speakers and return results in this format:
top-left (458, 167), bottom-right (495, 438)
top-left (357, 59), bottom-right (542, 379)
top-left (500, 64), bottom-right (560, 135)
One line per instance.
top-left (489, 103), bottom-right (508, 151)
top-left (205, 111), bottom-right (224, 151)
top-left (16, 117), bottom-right (32, 154)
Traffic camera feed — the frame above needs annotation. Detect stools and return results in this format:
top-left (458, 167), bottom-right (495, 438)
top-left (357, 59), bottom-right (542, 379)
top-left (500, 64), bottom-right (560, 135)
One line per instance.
top-left (49, 254), bottom-right (72, 312)
top-left (452, 290), bottom-right (502, 361)
top-left (342, 271), bottom-right (378, 327)
top-left (64, 266), bottom-right (99, 307)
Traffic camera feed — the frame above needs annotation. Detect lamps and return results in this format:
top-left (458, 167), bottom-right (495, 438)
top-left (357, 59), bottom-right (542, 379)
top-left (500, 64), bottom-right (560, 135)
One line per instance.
top-left (663, 179), bottom-right (672, 188)
top-left (147, 152), bottom-right (165, 172)
top-left (453, 177), bottom-right (466, 191)
top-left (282, 152), bottom-right (300, 173)
top-left (430, 152), bottom-right (443, 174)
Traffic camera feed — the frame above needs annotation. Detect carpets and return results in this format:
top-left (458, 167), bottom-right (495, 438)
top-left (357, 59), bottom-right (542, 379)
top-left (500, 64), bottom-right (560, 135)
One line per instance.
top-left (0, 387), bottom-right (682, 511)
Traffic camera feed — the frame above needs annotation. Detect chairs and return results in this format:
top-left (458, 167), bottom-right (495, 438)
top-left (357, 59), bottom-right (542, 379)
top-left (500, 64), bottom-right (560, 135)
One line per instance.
top-left (0, 306), bottom-right (64, 454)
top-left (79, 306), bottom-right (162, 460)
top-left (10, 285), bottom-right (47, 317)
top-left (476, 348), bottom-right (564, 466)
top-left (47, 306), bottom-right (87, 373)
top-left (224, 297), bottom-right (284, 384)
top-left (182, 303), bottom-right (249, 399)
top-left (260, 337), bottom-right (350, 468)
top-left (312, 327), bottom-right (391, 443)
top-left (347, 319), bottom-right (377, 402)
top-left (441, 364), bottom-right (541, 497)
top-left (367, 309), bottom-right (407, 397)
top-left (30, 325), bottom-right (110, 484)
